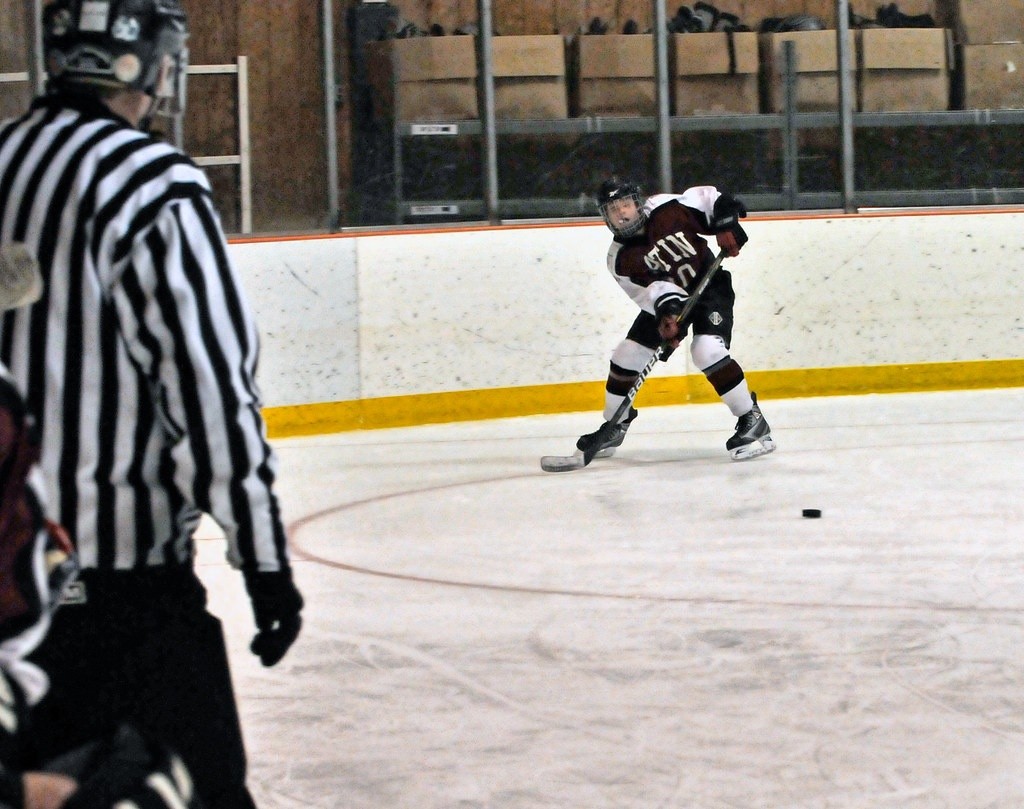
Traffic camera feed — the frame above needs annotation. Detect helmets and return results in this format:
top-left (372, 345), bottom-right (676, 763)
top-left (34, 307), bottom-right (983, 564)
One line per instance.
top-left (597, 175), bottom-right (649, 238)
top-left (42, 1), bottom-right (189, 97)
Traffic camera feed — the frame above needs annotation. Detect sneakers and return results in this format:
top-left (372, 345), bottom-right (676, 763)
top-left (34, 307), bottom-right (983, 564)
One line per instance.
top-left (577, 405), bottom-right (638, 458)
top-left (726, 391), bottom-right (775, 461)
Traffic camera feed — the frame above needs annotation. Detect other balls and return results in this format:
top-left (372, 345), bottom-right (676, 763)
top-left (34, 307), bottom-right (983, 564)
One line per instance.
top-left (801, 508), bottom-right (821, 518)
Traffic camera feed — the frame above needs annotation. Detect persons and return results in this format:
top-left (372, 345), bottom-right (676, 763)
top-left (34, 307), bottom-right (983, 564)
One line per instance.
top-left (577, 176), bottom-right (777, 461)
top-left (1, 1), bottom-right (306, 809)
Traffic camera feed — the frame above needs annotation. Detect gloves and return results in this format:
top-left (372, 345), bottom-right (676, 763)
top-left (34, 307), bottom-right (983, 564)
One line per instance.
top-left (713, 210), bottom-right (748, 257)
top-left (657, 302), bottom-right (687, 349)
top-left (242, 568), bottom-right (303, 666)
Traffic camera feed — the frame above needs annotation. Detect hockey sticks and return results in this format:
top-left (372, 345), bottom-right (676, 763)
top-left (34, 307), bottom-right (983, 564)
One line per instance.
top-left (540, 248), bottom-right (728, 474)
